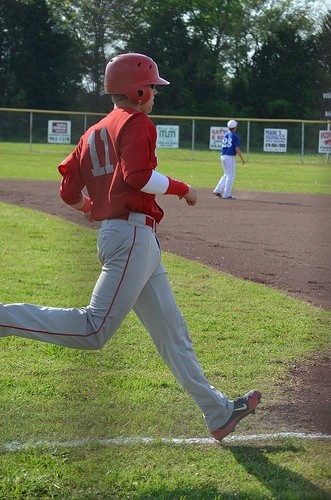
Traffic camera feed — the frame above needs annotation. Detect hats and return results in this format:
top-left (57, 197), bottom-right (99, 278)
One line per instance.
top-left (227, 120), bottom-right (237, 129)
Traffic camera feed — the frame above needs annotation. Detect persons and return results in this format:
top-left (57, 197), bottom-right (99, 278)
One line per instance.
top-left (0, 53), bottom-right (262, 441)
top-left (213, 120), bottom-right (246, 200)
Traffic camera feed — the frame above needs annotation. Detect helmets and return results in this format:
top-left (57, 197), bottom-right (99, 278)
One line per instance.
top-left (104, 54), bottom-right (170, 105)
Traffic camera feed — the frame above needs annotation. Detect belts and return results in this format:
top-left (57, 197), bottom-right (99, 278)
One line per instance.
top-left (113, 213), bottom-right (158, 234)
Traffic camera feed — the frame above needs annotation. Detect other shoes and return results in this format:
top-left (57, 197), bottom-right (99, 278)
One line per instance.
top-left (213, 192), bottom-right (222, 198)
top-left (228, 196), bottom-right (234, 199)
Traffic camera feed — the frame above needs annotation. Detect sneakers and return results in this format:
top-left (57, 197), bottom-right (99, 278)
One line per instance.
top-left (211, 389), bottom-right (262, 443)
